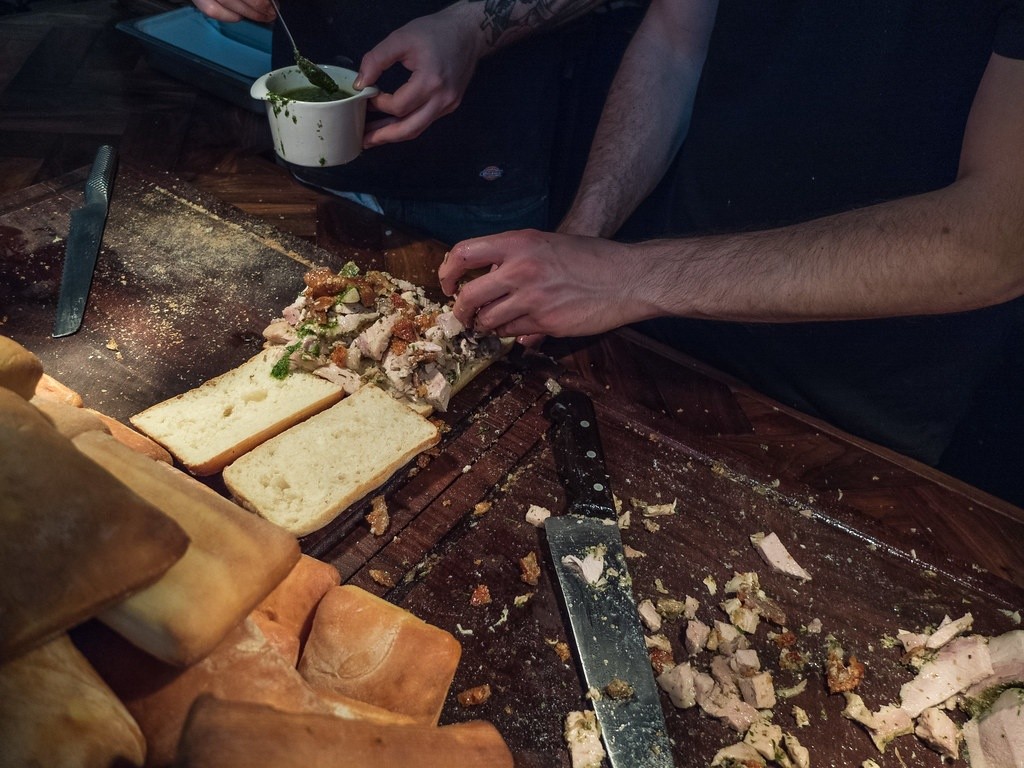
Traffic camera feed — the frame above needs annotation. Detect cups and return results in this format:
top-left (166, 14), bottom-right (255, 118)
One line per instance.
top-left (250, 64), bottom-right (379, 168)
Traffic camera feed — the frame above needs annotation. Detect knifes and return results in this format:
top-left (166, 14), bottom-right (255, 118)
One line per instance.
top-left (542, 388), bottom-right (673, 768)
top-left (50, 145), bottom-right (119, 341)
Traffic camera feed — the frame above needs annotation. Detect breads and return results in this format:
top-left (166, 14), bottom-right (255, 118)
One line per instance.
top-left (0, 321), bottom-right (521, 768)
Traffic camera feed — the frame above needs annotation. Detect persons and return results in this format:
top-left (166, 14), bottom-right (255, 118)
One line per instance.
top-left (438, 0), bottom-right (1024, 493)
top-left (193, 0), bottom-right (627, 248)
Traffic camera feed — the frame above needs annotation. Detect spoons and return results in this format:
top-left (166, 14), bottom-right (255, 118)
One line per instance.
top-left (268, 0), bottom-right (339, 93)
